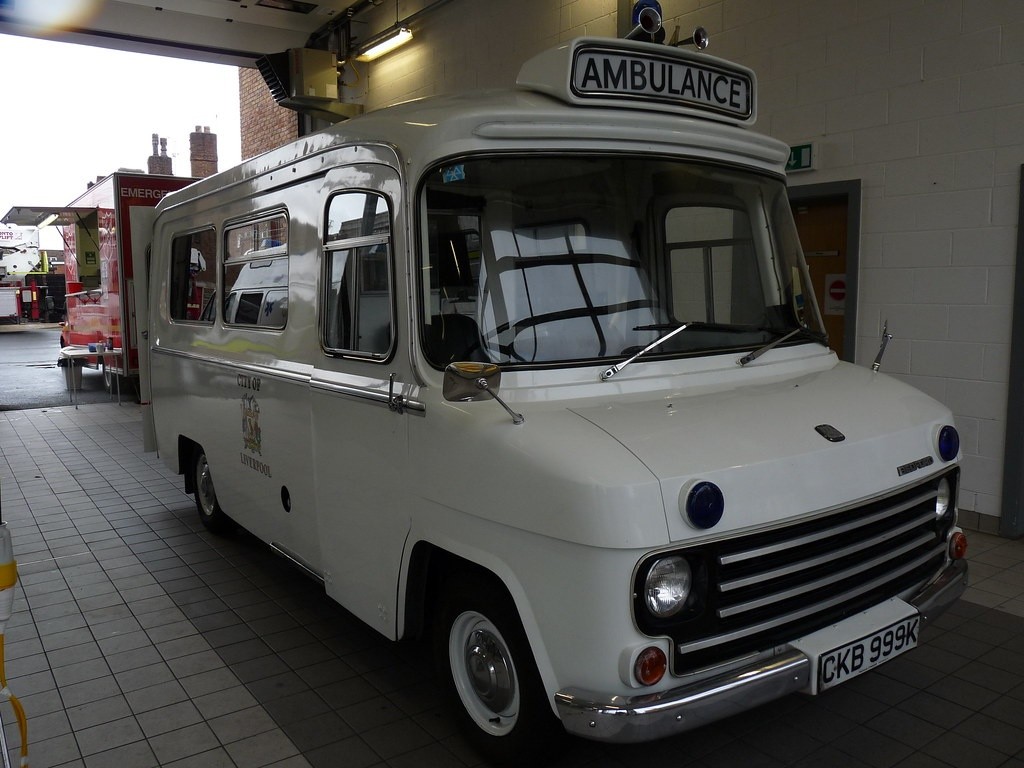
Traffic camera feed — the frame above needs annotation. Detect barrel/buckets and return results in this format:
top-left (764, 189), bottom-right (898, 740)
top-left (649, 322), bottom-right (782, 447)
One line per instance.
top-left (67, 283), bottom-right (83, 293)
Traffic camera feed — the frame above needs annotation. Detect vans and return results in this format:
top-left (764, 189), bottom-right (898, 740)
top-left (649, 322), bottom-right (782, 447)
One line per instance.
top-left (148, 6), bottom-right (972, 768)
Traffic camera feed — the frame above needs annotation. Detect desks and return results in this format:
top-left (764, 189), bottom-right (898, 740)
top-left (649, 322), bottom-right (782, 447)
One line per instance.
top-left (58, 344), bottom-right (123, 410)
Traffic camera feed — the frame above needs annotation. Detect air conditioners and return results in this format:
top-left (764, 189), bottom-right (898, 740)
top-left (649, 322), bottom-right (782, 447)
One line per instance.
top-left (268, 47), bottom-right (339, 101)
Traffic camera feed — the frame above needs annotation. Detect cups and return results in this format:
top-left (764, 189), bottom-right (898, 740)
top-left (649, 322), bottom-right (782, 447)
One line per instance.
top-left (96, 345), bottom-right (104, 353)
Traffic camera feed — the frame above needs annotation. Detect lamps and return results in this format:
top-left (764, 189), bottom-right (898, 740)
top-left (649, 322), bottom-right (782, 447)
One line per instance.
top-left (343, 0), bottom-right (416, 62)
